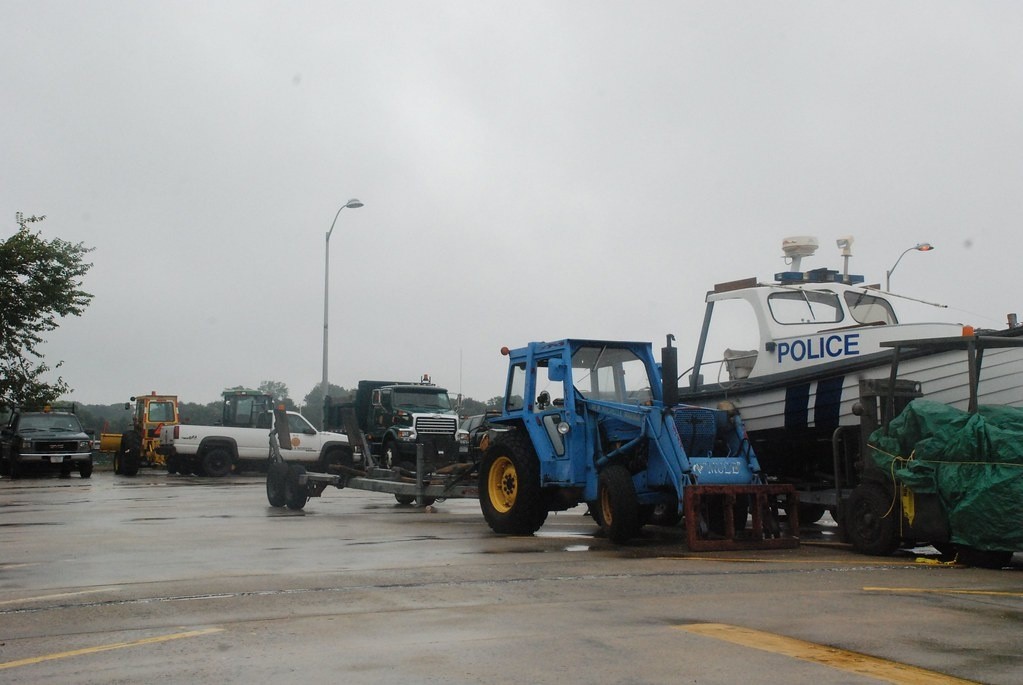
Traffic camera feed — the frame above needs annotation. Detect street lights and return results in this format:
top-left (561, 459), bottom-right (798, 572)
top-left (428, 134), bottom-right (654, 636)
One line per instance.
top-left (885, 241), bottom-right (935, 292)
top-left (318, 197), bottom-right (365, 431)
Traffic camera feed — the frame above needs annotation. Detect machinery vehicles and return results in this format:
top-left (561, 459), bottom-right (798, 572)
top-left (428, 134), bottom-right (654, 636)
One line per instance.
top-left (577, 235), bottom-right (1023, 568)
top-left (265, 332), bottom-right (800, 552)
top-left (98, 389), bottom-right (180, 477)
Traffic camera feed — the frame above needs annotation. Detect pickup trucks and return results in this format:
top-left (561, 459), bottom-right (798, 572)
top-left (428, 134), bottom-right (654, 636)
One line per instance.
top-left (153, 409), bottom-right (363, 478)
top-left (0, 404), bottom-right (93, 478)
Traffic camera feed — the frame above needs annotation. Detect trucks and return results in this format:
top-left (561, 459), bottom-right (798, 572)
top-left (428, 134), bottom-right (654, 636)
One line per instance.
top-left (320, 375), bottom-right (458, 471)
